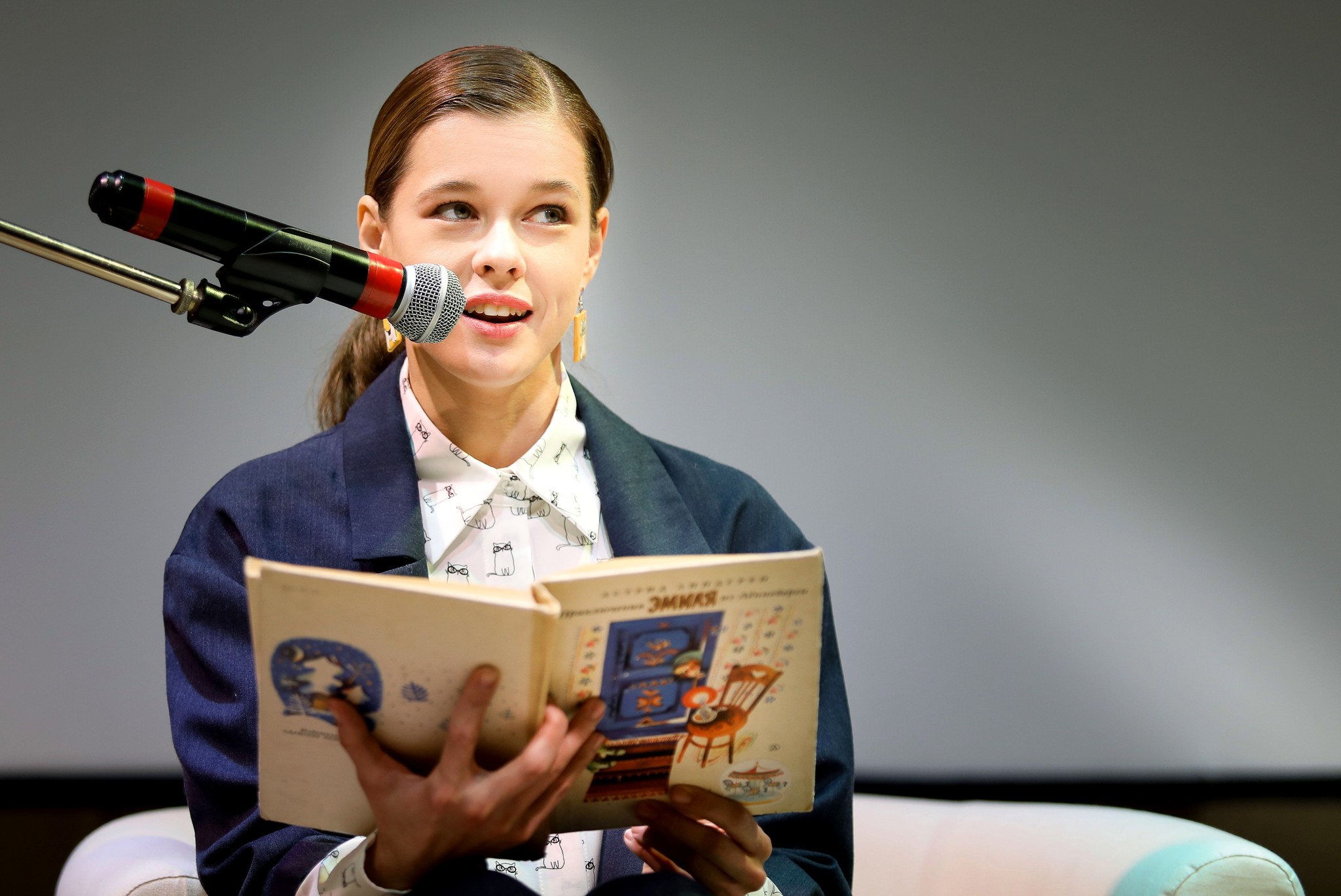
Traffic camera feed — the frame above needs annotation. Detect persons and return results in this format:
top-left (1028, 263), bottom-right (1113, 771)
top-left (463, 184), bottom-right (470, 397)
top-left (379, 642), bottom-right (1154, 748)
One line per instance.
top-left (159, 46), bottom-right (855, 896)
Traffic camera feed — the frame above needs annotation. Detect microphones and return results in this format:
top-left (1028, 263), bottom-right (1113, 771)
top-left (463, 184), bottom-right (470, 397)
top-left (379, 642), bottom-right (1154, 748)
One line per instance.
top-left (88, 170), bottom-right (466, 343)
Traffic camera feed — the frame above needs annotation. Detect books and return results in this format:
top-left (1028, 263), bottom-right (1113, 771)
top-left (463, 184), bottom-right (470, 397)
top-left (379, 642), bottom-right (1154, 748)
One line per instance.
top-left (243, 547), bottom-right (826, 840)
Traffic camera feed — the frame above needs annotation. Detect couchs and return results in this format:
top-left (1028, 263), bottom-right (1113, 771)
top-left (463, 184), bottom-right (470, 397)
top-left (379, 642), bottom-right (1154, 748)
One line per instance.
top-left (57, 792), bottom-right (1303, 894)
